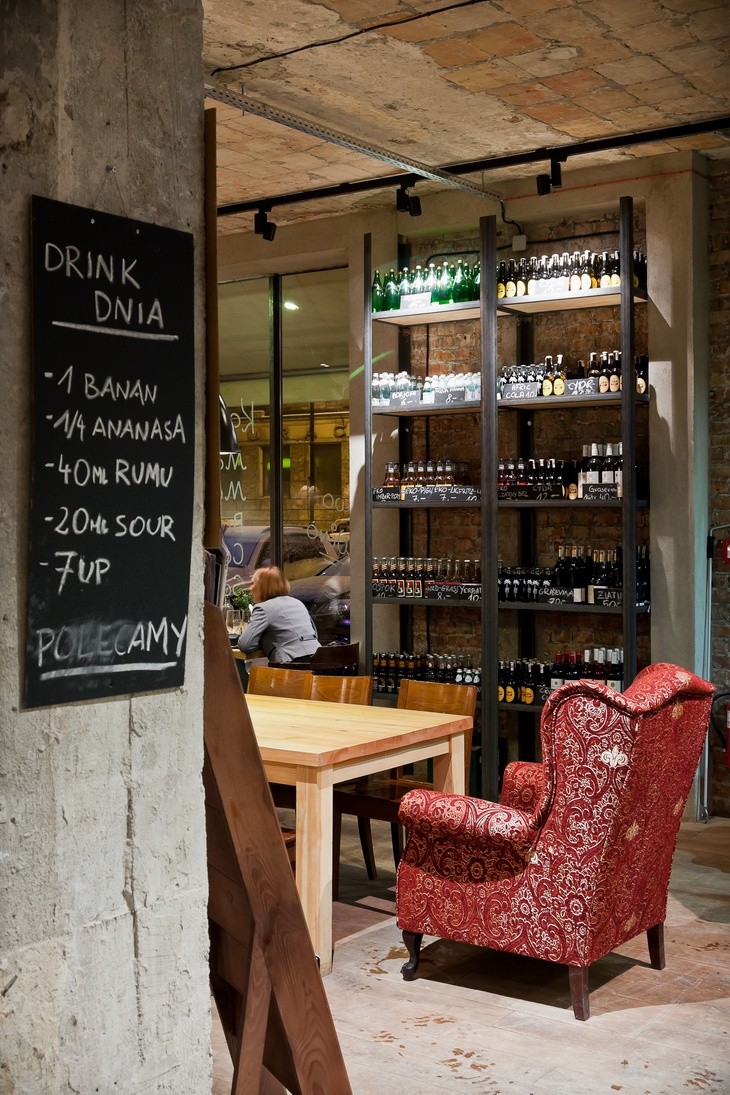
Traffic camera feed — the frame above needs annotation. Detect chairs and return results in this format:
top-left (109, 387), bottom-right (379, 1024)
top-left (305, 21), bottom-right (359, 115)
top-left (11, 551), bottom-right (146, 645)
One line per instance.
top-left (394, 662), bottom-right (718, 1020)
top-left (333, 677), bottom-right (477, 899)
top-left (312, 676), bottom-right (380, 885)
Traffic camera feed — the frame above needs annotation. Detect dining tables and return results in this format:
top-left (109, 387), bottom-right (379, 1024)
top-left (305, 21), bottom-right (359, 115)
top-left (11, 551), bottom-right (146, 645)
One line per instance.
top-left (240, 693), bottom-right (473, 979)
top-left (246, 663), bottom-right (313, 697)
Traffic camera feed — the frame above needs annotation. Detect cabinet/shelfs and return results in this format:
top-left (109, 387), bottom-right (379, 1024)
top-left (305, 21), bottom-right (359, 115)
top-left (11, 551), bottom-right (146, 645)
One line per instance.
top-left (360, 191), bottom-right (652, 803)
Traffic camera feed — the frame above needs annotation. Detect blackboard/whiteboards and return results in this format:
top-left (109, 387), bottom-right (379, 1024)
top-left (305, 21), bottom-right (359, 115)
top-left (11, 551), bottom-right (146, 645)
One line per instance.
top-left (13, 190), bottom-right (196, 716)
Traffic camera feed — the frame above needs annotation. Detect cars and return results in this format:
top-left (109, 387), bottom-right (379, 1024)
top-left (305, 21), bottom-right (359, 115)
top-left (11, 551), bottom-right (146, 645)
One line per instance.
top-left (287, 550), bottom-right (350, 644)
top-left (327, 518), bottom-right (350, 552)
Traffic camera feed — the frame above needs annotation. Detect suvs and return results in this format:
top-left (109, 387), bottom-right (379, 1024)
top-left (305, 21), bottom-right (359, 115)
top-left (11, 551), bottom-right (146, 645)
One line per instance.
top-left (217, 524), bottom-right (342, 604)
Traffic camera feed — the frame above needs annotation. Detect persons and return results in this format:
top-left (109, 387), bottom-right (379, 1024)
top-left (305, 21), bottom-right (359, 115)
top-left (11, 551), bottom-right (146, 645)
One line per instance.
top-left (236, 566), bottom-right (322, 664)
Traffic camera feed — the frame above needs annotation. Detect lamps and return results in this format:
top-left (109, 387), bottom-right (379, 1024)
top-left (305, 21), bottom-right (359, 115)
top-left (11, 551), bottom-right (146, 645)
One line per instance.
top-left (394, 171), bottom-right (423, 217)
top-left (254, 197), bottom-right (277, 239)
top-left (537, 152), bottom-right (568, 196)
top-left (218, 390), bottom-right (241, 455)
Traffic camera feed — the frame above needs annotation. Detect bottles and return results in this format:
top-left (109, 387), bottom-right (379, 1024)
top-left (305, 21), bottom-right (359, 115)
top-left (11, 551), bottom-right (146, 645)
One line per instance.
top-left (365, 248), bottom-right (650, 707)
top-left (222, 594), bottom-right (233, 621)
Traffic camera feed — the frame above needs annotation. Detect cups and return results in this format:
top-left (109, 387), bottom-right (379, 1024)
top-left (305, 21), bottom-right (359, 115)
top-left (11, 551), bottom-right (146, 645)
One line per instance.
top-left (226, 610), bottom-right (241, 635)
top-left (241, 610), bottom-right (252, 635)
